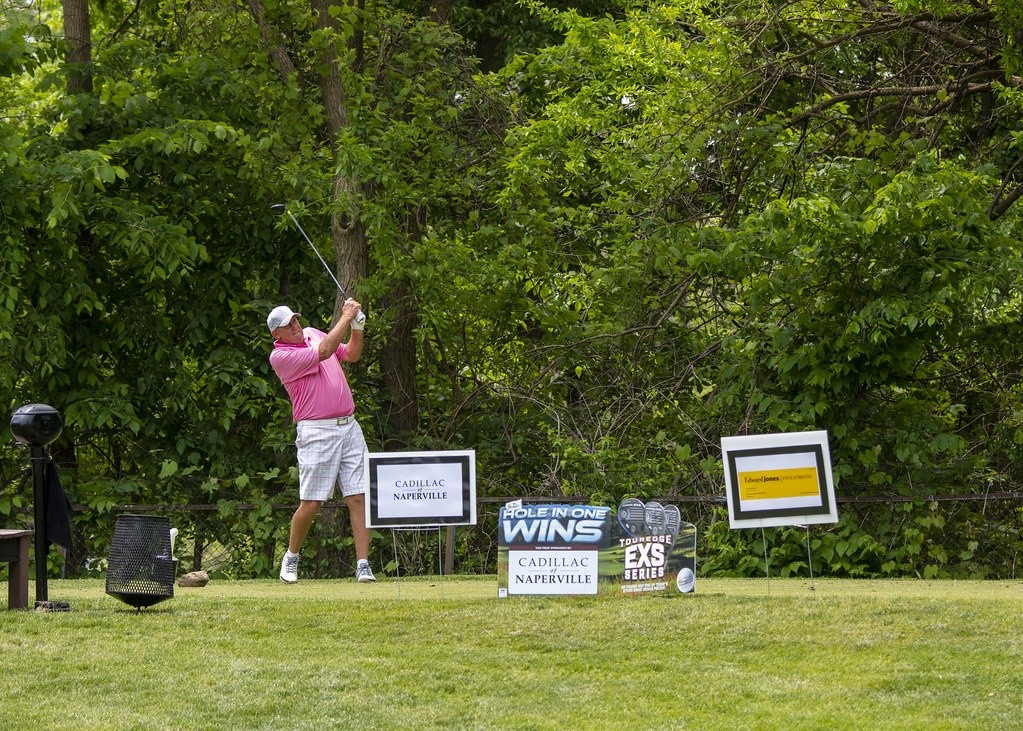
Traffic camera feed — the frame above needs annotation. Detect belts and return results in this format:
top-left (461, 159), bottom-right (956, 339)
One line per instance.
top-left (297, 414), bottom-right (354, 426)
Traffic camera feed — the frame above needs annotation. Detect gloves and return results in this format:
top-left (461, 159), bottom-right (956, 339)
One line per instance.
top-left (349, 308), bottom-right (366, 330)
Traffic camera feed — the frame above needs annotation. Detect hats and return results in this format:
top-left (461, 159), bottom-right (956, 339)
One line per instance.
top-left (267, 305), bottom-right (302, 334)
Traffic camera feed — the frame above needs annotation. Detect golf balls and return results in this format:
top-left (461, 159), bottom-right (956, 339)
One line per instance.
top-left (675, 568), bottom-right (694, 593)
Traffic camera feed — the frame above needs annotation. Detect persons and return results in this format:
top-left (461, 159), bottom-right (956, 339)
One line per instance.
top-left (269, 297), bottom-right (375, 581)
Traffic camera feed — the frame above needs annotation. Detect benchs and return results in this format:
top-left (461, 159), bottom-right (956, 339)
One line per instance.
top-left (0, 529), bottom-right (34, 612)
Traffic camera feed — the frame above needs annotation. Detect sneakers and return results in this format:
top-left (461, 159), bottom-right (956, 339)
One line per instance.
top-left (280, 552), bottom-right (301, 584)
top-left (355, 561), bottom-right (376, 582)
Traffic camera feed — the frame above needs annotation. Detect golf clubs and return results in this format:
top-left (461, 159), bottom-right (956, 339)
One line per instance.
top-left (271, 204), bottom-right (349, 300)
